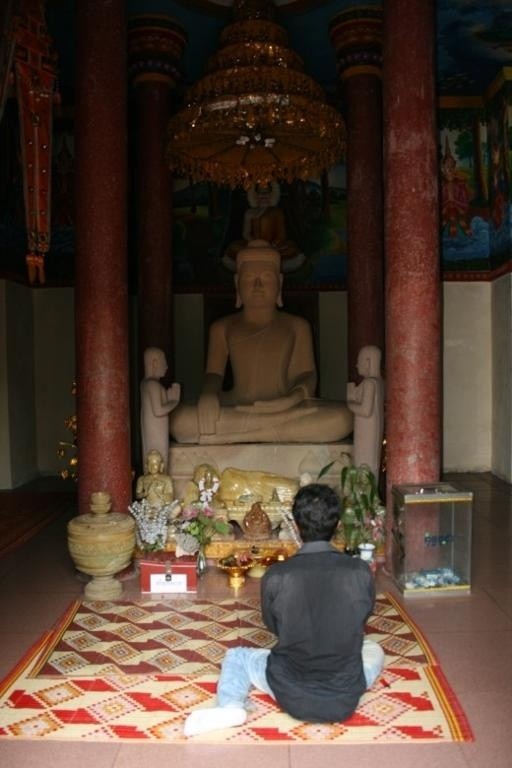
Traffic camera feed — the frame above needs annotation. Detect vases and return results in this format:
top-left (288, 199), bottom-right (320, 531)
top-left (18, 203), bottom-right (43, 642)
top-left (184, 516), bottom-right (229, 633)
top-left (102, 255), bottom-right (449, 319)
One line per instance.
top-left (196, 545), bottom-right (207, 575)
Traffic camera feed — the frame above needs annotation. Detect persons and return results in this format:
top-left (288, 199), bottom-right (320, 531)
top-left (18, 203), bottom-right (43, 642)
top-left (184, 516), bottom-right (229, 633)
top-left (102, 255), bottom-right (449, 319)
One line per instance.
top-left (489, 145), bottom-right (507, 225)
top-left (227, 178), bottom-right (298, 260)
top-left (135, 449), bottom-right (176, 509)
top-left (139, 347), bottom-right (180, 474)
top-left (442, 155), bottom-right (473, 234)
top-left (344, 344), bottom-right (386, 485)
top-left (168, 241), bottom-right (354, 443)
top-left (181, 481), bottom-right (385, 739)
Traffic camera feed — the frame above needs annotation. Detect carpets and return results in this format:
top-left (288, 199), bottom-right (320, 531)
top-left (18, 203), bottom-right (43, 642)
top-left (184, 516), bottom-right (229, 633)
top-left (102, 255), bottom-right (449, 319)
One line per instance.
top-left (2, 593), bottom-right (476, 745)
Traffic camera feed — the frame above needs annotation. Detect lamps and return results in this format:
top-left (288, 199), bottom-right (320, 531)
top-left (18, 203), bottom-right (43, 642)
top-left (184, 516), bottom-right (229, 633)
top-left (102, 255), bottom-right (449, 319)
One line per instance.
top-left (163, 1), bottom-right (352, 194)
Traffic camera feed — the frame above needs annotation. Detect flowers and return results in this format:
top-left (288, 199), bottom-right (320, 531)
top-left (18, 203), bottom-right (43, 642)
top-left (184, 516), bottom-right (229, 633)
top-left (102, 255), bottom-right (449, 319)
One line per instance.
top-left (126, 497), bottom-right (182, 552)
top-left (179, 502), bottom-right (230, 544)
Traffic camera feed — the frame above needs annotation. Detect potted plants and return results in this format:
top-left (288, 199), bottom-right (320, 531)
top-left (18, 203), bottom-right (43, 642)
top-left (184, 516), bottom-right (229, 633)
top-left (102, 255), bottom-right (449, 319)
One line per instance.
top-left (316, 450), bottom-right (383, 562)
